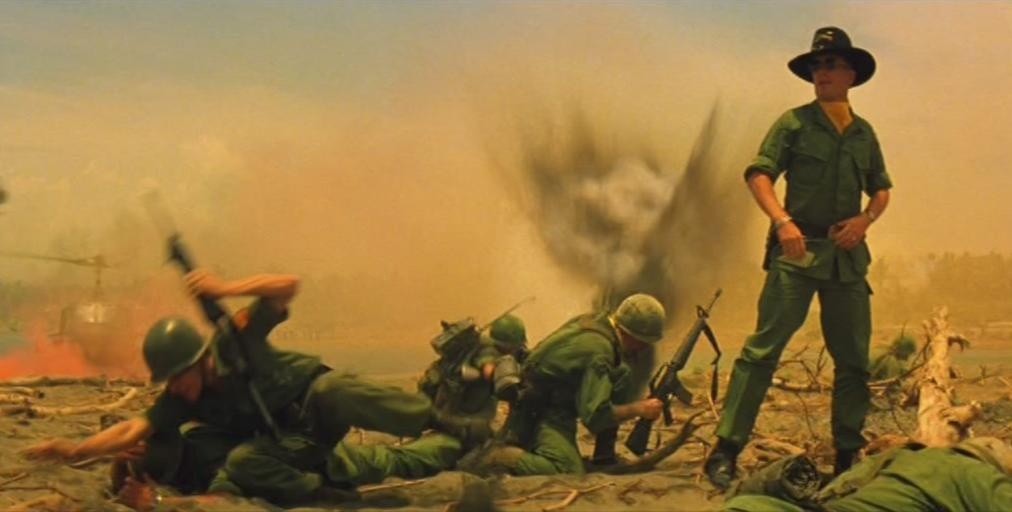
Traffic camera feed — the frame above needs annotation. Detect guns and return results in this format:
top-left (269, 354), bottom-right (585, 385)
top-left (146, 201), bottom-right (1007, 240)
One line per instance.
top-left (624, 287), bottom-right (722, 455)
top-left (168, 230), bottom-right (275, 433)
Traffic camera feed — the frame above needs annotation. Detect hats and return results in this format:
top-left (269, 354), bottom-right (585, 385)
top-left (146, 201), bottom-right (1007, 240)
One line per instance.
top-left (789, 28), bottom-right (876, 86)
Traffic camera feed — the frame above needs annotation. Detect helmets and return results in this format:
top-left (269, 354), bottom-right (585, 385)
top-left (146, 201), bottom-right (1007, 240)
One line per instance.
top-left (488, 316), bottom-right (527, 347)
top-left (951, 435), bottom-right (1011, 473)
top-left (142, 319), bottom-right (215, 382)
top-left (612, 294), bottom-right (667, 343)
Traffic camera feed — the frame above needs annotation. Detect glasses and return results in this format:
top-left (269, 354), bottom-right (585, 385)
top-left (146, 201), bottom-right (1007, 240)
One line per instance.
top-left (803, 56), bottom-right (841, 70)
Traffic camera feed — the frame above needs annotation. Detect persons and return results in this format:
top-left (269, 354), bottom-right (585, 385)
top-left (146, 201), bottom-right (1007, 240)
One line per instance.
top-left (868, 333), bottom-right (921, 413)
top-left (20, 272), bottom-right (668, 512)
top-left (729, 434), bottom-right (1012, 511)
top-left (704, 27), bottom-right (893, 491)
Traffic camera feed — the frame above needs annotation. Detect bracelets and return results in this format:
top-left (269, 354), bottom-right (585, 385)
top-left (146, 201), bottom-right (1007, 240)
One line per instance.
top-left (772, 213), bottom-right (793, 229)
top-left (863, 209), bottom-right (876, 224)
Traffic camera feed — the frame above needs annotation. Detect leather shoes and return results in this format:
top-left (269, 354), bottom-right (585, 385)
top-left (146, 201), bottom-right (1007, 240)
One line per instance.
top-left (705, 442), bottom-right (735, 487)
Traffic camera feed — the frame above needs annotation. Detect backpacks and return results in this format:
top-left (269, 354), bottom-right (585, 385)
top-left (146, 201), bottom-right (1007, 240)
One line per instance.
top-left (431, 319), bottom-right (481, 361)
